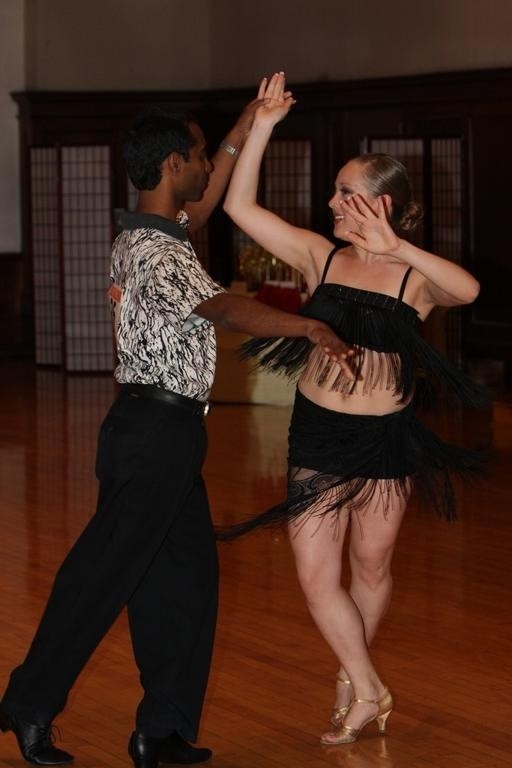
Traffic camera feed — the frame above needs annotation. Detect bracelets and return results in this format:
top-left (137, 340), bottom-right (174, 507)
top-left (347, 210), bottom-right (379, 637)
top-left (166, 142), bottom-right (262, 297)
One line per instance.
top-left (222, 140), bottom-right (239, 157)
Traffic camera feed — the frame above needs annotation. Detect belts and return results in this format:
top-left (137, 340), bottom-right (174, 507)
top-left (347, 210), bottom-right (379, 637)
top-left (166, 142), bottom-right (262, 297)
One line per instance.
top-left (128, 383), bottom-right (212, 417)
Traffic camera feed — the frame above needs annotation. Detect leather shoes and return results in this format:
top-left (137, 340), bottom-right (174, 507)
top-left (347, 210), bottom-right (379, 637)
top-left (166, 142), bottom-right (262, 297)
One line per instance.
top-left (128, 731), bottom-right (213, 768)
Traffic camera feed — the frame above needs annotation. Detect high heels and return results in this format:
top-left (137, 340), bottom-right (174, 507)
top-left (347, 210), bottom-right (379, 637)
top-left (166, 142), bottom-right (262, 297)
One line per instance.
top-left (319, 673), bottom-right (394, 745)
top-left (0, 701), bottom-right (75, 766)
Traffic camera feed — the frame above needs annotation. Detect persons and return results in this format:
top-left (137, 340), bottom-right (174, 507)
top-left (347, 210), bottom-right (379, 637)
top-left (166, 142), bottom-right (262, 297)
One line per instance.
top-left (221, 70), bottom-right (481, 745)
top-left (0, 90), bottom-right (358, 768)
